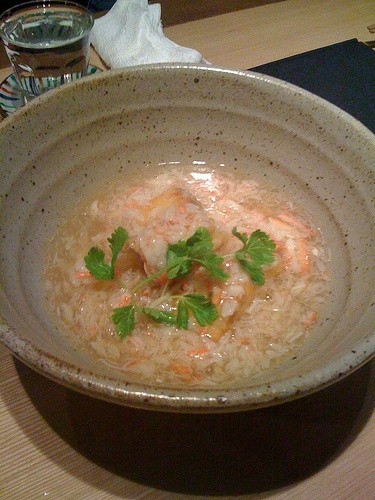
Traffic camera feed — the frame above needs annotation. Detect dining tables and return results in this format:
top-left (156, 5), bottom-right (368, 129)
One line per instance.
top-left (0, 0), bottom-right (374, 499)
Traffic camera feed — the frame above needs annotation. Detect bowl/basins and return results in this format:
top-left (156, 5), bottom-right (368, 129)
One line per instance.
top-left (0, 63), bottom-right (375, 415)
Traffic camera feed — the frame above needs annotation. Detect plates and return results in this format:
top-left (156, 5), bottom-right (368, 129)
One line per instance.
top-left (0, 64), bottom-right (104, 116)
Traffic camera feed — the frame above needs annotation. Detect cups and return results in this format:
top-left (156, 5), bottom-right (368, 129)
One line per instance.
top-left (1, 1), bottom-right (95, 103)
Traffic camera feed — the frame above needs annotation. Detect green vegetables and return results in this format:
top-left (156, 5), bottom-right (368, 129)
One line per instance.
top-left (82, 223), bottom-right (277, 337)
